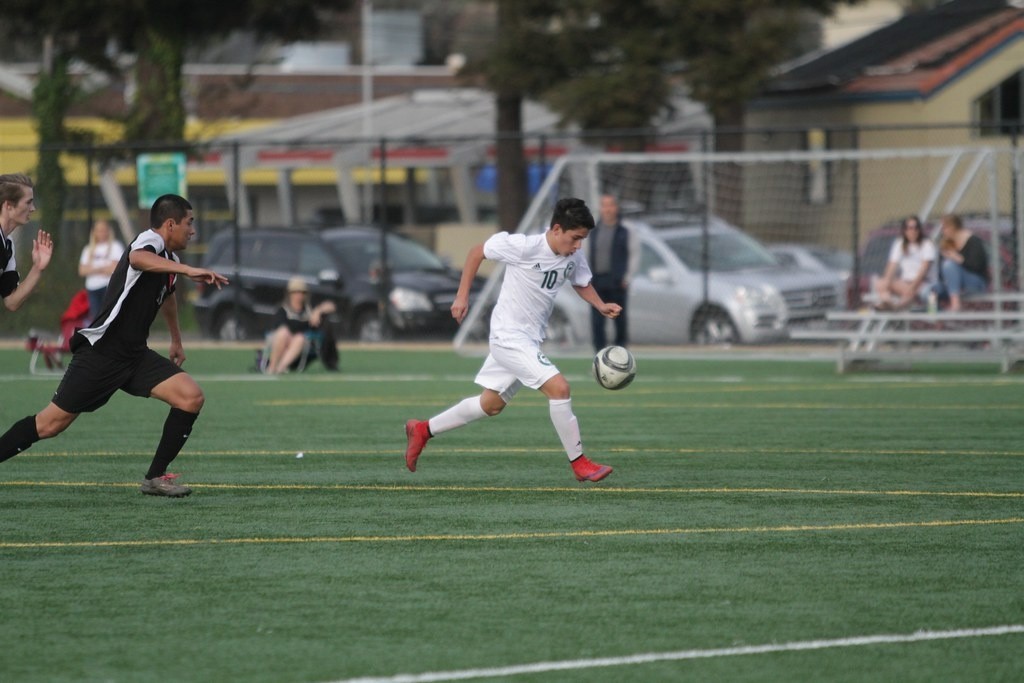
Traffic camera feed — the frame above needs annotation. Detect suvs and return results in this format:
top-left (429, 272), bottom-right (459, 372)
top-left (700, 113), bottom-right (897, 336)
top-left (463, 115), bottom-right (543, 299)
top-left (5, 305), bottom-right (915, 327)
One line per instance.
top-left (195, 225), bottom-right (496, 343)
top-left (854, 214), bottom-right (1023, 354)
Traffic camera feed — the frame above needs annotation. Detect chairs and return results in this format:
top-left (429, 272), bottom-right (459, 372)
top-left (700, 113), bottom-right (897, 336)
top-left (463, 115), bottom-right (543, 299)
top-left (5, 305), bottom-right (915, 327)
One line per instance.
top-left (25, 320), bottom-right (86, 376)
top-left (258, 329), bottom-right (323, 375)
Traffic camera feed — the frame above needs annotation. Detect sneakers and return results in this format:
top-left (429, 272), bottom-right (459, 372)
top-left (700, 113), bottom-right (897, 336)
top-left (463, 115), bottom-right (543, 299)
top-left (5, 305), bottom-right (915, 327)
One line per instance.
top-left (142, 472), bottom-right (192, 497)
top-left (574, 458), bottom-right (612, 482)
top-left (406, 418), bottom-right (428, 472)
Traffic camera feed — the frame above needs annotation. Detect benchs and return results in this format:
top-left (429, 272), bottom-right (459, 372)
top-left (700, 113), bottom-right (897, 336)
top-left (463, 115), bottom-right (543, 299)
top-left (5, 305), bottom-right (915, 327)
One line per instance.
top-left (787, 291), bottom-right (1024, 374)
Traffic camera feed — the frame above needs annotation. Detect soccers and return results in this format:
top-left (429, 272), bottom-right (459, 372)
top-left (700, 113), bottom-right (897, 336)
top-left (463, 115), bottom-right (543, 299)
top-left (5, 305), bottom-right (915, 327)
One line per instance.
top-left (592, 346), bottom-right (637, 391)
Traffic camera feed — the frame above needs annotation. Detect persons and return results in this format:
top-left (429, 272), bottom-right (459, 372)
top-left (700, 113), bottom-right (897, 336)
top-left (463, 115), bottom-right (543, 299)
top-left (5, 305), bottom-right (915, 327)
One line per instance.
top-left (587, 196), bottom-right (636, 353)
top-left (873, 215), bottom-right (939, 311)
top-left (0, 172), bottom-right (54, 312)
top-left (920, 215), bottom-right (990, 311)
top-left (406, 196), bottom-right (622, 481)
top-left (77, 221), bottom-right (126, 320)
top-left (267, 274), bottom-right (335, 375)
top-left (1, 194), bottom-right (228, 498)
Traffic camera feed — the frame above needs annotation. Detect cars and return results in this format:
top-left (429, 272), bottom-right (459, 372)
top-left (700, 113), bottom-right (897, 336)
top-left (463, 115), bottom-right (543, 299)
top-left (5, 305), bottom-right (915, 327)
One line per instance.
top-left (460, 216), bottom-right (848, 350)
top-left (762, 245), bottom-right (847, 276)
